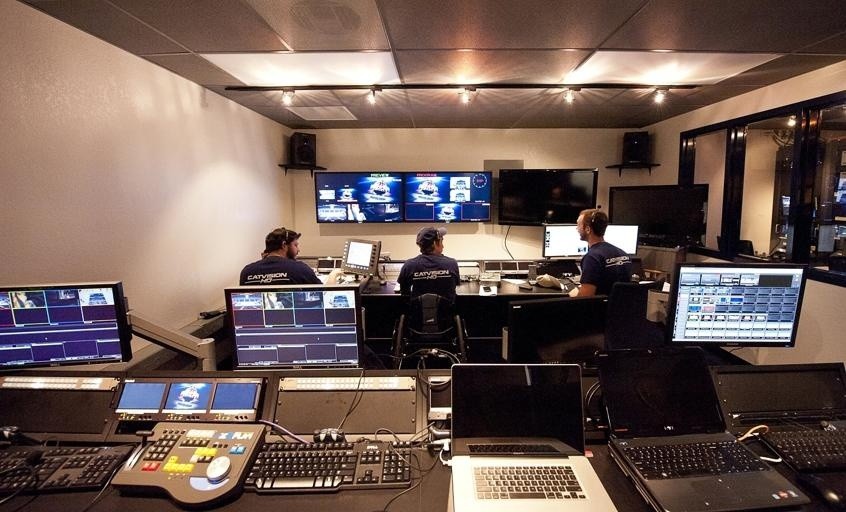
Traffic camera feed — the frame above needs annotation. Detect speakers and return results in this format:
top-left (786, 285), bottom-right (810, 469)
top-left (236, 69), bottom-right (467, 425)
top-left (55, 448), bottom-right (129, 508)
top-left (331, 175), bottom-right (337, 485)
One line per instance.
top-left (290, 132), bottom-right (316, 167)
top-left (622, 132), bottom-right (650, 164)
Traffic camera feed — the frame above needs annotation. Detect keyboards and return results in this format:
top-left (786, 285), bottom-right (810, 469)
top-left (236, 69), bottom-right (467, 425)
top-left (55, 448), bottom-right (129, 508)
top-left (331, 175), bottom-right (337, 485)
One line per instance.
top-left (0, 446), bottom-right (134, 494)
top-left (244, 442), bottom-right (411, 494)
top-left (760, 429), bottom-right (846, 472)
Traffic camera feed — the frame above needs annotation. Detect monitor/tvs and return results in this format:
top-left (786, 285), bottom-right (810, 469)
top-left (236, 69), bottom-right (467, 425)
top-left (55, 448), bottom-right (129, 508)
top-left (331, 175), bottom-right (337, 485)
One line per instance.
top-left (543, 223), bottom-right (639, 258)
top-left (608, 184), bottom-right (709, 248)
top-left (314, 168), bottom-right (493, 223)
top-left (341, 239), bottom-right (381, 275)
top-left (498, 170), bottom-right (599, 227)
top-left (224, 284), bottom-right (364, 372)
top-left (665, 263), bottom-right (808, 347)
top-left (0, 280), bottom-right (217, 371)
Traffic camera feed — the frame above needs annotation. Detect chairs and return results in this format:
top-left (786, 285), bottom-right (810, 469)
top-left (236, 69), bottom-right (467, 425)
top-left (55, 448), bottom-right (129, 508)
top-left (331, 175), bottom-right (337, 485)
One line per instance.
top-left (603, 276), bottom-right (665, 354)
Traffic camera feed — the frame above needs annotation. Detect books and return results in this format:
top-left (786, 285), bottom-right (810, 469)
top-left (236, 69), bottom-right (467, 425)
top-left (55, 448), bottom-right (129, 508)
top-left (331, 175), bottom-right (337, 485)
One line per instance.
top-left (479, 272), bottom-right (501, 288)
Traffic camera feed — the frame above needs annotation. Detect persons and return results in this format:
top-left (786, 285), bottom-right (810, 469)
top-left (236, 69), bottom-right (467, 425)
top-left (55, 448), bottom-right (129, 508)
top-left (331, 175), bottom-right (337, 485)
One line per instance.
top-left (222, 226), bottom-right (347, 356)
top-left (396, 226), bottom-right (462, 343)
top-left (535, 208), bottom-right (633, 360)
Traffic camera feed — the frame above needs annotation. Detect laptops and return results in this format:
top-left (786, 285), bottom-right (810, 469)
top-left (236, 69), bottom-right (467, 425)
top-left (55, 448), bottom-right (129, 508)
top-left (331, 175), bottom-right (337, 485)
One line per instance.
top-left (451, 363), bottom-right (619, 512)
top-left (595, 347), bottom-right (812, 512)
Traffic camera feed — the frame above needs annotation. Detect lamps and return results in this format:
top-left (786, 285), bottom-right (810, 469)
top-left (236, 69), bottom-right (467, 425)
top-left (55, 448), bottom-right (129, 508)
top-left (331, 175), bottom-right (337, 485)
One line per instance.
top-left (224, 85), bottom-right (704, 104)
